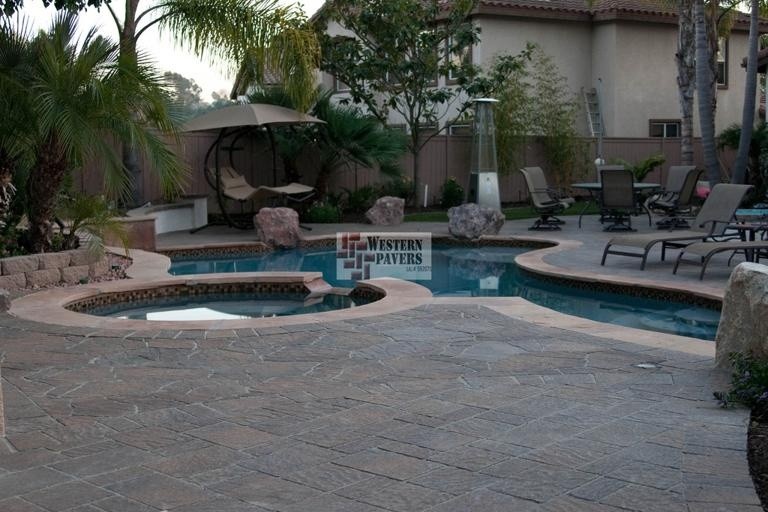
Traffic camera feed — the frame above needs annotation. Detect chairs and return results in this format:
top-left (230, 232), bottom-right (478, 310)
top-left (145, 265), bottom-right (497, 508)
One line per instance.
top-left (204, 166), bottom-right (319, 233)
top-left (519, 165), bottom-right (768, 280)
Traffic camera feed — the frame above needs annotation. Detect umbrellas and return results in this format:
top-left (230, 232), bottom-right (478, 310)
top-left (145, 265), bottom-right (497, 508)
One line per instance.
top-left (186, 298), bottom-right (324, 318)
top-left (163, 100), bottom-right (329, 133)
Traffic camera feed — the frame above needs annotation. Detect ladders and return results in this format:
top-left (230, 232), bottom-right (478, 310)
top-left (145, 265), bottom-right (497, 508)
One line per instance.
top-left (581, 87), bottom-right (604, 137)
